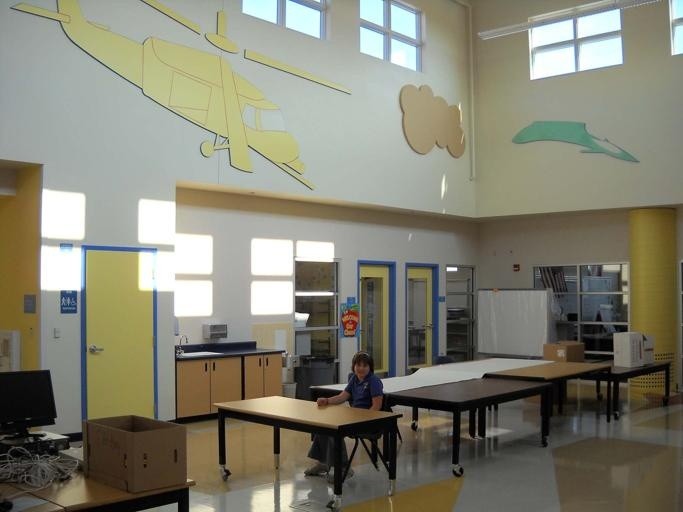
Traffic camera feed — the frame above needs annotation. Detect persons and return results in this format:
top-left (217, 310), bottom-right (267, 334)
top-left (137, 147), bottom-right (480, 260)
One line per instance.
top-left (303, 351), bottom-right (383, 484)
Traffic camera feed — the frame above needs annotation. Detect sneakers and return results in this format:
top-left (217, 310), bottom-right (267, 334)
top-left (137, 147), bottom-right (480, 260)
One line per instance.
top-left (326, 467), bottom-right (354, 482)
top-left (305, 463), bottom-right (328, 476)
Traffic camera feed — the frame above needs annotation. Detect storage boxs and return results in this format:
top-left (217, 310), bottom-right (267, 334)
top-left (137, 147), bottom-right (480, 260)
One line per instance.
top-left (82, 414), bottom-right (187, 494)
top-left (644, 336), bottom-right (655, 364)
top-left (613, 332), bottom-right (645, 369)
top-left (543, 340), bottom-right (585, 362)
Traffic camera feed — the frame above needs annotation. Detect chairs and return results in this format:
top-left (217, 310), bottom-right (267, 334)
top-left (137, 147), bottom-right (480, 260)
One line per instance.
top-left (340, 394), bottom-right (393, 482)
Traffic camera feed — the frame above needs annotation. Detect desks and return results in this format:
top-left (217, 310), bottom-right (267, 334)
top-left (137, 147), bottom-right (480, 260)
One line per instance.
top-left (483, 362), bottom-right (613, 423)
top-left (0, 482), bottom-right (66, 512)
top-left (5, 471), bottom-right (196, 512)
top-left (215, 395), bottom-right (405, 510)
top-left (578, 359), bottom-right (672, 421)
top-left (412, 357), bottom-right (547, 378)
top-left (390, 377), bottom-right (552, 477)
top-left (310, 374), bottom-right (482, 439)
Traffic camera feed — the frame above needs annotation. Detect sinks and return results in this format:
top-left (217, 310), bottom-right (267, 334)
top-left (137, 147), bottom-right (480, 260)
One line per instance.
top-left (176, 352), bottom-right (224, 356)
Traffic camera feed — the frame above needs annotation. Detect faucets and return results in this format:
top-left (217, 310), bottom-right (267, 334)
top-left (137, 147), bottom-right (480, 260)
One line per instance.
top-left (179, 335), bottom-right (188, 347)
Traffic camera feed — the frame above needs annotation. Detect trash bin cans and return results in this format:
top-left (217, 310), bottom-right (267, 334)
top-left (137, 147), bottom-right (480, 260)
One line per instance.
top-left (280, 382), bottom-right (296, 398)
top-left (296, 354), bottom-right (335, 402)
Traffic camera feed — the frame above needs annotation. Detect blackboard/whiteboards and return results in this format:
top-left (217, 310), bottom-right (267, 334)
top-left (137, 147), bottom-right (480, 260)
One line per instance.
top-left (477, 288), bottom-right (551, 357)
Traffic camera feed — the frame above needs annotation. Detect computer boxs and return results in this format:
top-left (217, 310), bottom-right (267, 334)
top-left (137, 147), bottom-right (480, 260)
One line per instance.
top-left (0, 431), bottom-right (70, 458)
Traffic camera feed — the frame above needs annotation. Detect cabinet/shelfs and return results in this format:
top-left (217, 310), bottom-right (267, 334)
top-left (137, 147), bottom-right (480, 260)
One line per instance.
top-left (176, 356), bottom-right (242, 419)
top-left (244, 353), bottom-right (283, 401)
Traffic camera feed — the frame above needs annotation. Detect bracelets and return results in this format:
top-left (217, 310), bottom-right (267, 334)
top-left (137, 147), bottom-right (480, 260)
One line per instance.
top-left (326, 398), bottom-right (328, 405)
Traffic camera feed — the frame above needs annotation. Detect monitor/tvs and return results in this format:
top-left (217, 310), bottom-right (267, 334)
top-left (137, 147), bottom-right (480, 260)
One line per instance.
top-left (0, 370), bottom-right (57, 440)
